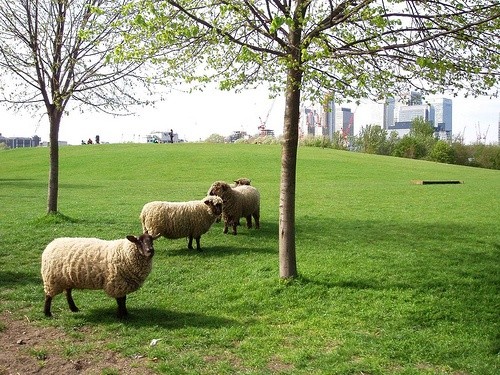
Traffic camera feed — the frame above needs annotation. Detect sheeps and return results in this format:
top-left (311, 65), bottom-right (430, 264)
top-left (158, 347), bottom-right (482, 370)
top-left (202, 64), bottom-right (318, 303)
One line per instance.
top-left (206, 178), bottom-right (261, 235)
top-left (140, 195), bottom-right (223, 251)
top-left (40, 232), bottom-right (161, 316)
